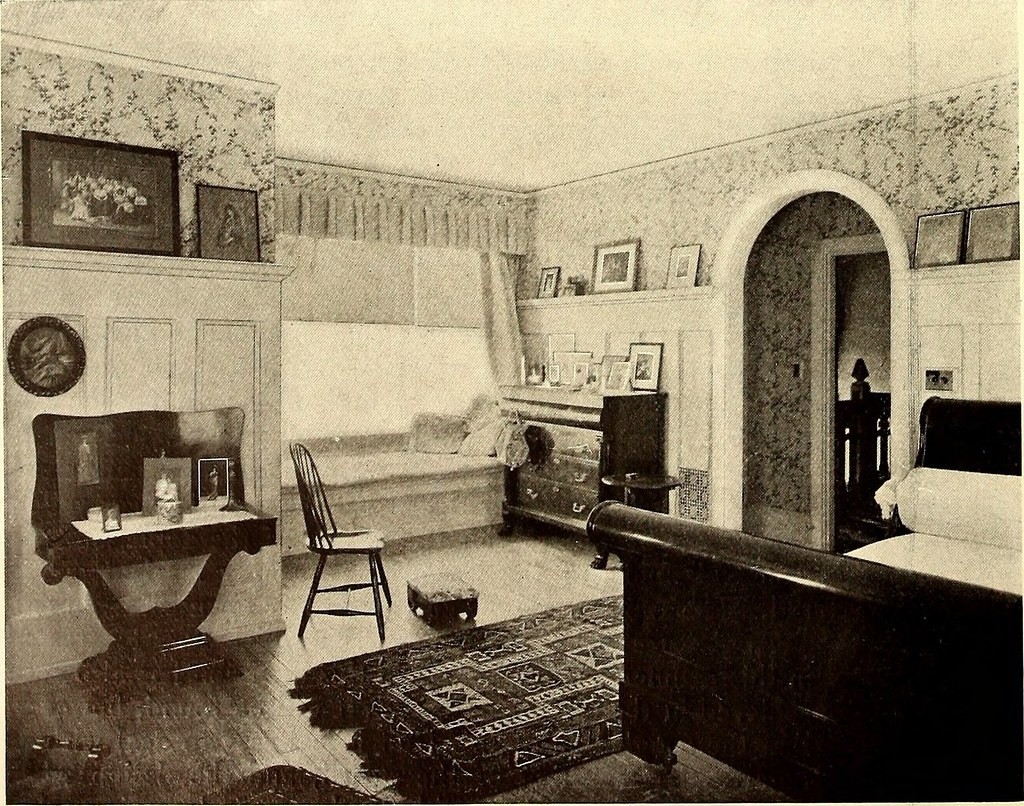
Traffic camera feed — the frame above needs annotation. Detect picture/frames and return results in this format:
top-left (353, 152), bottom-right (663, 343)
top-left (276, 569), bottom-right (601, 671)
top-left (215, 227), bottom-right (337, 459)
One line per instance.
top-left (551, 351), bottom-right (594, 387)
top-left (601, 355), bottom-right (628, 377)
top-left (590, 238), bottom-right (640, 293)
top-left (7, 316), bottom-right (87, 398)
top-left (194, 182), bottom-right (261, 263)
top-left (628, 343), bottom-right (664, 391)
top-left (912, 210), bottom-right (966, 268)
top-left (101, 503), bottom-right (122, 533)
top-left (965, 201), bottom-right (1020, 263)
top-left (193, 456), bottom-right (237, 509)
top-left (536, 266), bottom-right (560, 299)
top-left (142, 458), bottom-right (192, 516)
top-left (22, 130), bottom-right (182, 259)
top-left (606, 361), bottom-right (632, 389)
top-left (665, 243), bottom-right (701, 289)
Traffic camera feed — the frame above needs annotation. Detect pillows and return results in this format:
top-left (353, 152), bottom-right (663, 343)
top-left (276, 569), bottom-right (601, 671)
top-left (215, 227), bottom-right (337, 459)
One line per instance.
top-left (408, 412), bottom-right (470, 454)
top-left (874, 467), bottom-right (1024, 549)
top-left (459, 394), bottom-right (505, 456)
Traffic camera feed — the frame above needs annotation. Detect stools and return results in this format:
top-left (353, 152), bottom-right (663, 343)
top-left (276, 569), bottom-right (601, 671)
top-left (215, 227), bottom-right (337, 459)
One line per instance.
top-left (407, 572), bottom-right (481, 627)
top-left (285, 595), bottom-right (623, 804)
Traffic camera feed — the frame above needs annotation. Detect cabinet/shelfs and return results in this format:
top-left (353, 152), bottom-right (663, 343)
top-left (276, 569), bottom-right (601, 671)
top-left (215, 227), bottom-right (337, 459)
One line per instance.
top-left (498, 385), bottom-right (668, 569)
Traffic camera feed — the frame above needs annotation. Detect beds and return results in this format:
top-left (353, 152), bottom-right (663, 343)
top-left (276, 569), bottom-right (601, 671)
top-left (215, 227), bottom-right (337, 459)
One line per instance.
top-left (586, 397), bottom-right (1024, 802)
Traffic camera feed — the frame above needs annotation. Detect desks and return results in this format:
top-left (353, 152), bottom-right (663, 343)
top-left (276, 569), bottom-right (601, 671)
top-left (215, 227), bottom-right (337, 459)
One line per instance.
top-left (35, 502), bottom-right (279, 706)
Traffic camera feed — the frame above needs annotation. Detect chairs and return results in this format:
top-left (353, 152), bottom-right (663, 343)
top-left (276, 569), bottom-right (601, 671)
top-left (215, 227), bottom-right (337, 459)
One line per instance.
top-left (288, 442), bottom-right (392, 643)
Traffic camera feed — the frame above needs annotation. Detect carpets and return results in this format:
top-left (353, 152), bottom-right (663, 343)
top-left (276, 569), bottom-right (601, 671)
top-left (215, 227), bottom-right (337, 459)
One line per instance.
top-left (189, 765), bottom-right (392, 805)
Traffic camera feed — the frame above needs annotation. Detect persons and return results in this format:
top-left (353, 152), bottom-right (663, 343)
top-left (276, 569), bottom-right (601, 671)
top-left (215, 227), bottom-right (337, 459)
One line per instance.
top-left (636, 362), bottom-right (651, 379)
top-left (154, 470), bottom-right (177, 505)
top-left (219, 204), bottom-right (243, 248)
top-left (208, 465), bottom-right (219, 499)
top-left (62, 171), bottom-right (147, 224)
top-left (78, 435), bottom-right (96, 484)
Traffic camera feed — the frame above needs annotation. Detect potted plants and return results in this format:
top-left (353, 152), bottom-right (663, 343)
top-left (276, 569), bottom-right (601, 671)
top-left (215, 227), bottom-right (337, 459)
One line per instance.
top-left (562, 275), bottom-right (588, 296)
